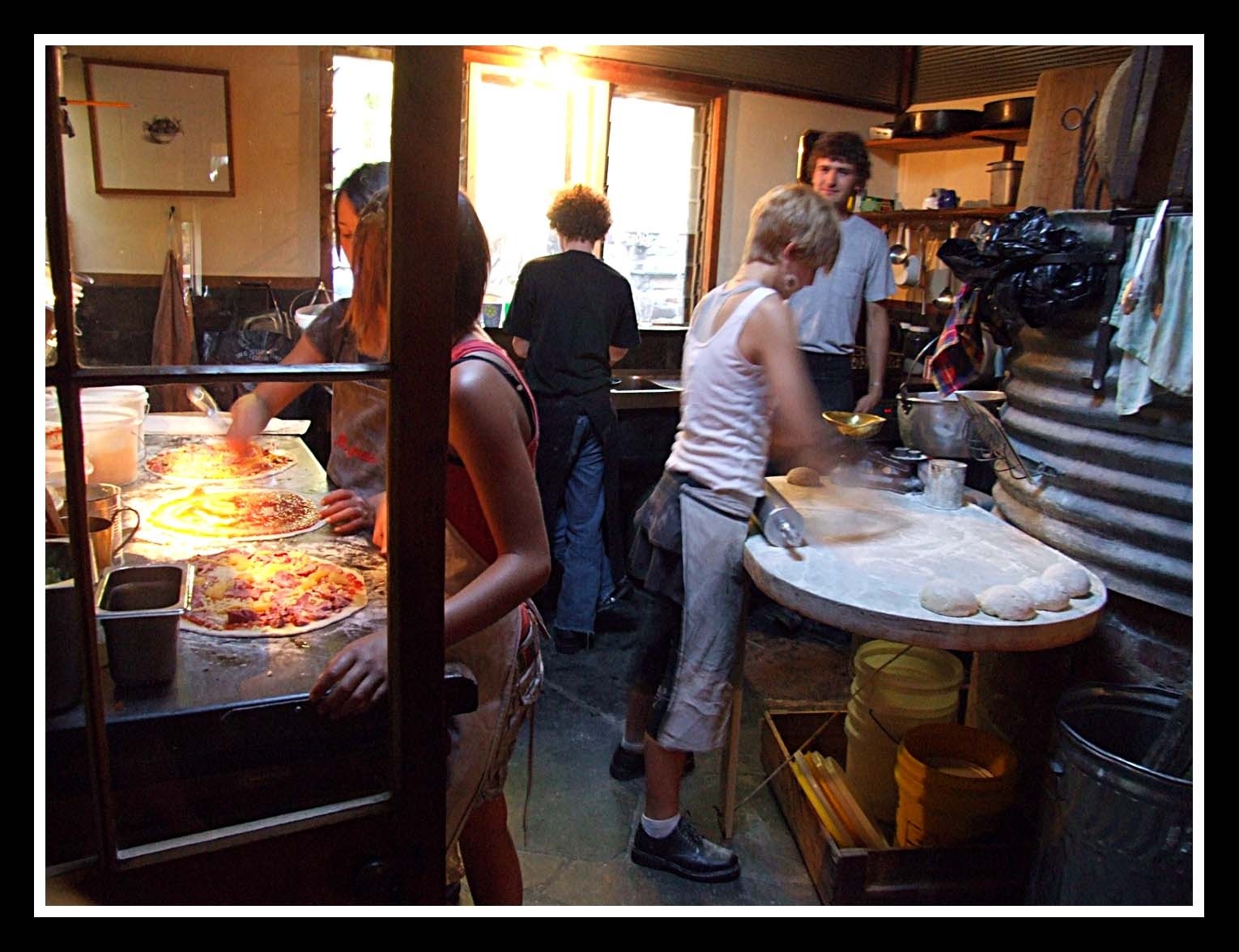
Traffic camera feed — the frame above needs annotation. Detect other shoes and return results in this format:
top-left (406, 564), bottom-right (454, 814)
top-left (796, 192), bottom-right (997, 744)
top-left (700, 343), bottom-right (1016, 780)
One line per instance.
top-left (554, 631), bottom-right (593, 653)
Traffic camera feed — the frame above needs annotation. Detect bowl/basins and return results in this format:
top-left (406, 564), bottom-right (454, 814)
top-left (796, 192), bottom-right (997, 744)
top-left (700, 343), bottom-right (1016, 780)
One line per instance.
top-left (891, 228), bottom-right (919, 287)
top-left (822, 411), bottom-right (887, 439)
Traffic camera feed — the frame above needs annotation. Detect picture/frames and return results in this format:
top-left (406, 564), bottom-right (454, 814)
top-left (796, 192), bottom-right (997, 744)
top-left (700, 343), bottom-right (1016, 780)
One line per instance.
top-left (82, 56), bottom-right (236, 198)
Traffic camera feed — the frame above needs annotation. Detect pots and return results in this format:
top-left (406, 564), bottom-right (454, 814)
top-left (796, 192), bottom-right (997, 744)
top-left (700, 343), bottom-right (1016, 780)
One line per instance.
top-left (894, 110), bottom-right (983, 138)
top-left (985, 161), bottom-right (1024, 207)
top-left (895, 335), bottom-right (1007, 460)
top-left (984, 96), bottom-right (1035, 129)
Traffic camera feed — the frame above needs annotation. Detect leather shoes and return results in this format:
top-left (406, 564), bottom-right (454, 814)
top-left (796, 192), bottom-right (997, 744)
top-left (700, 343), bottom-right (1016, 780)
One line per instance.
top-left (630, 816), bottom-right (742, 884)
top-left (609, 745), bottom-right (695, 779)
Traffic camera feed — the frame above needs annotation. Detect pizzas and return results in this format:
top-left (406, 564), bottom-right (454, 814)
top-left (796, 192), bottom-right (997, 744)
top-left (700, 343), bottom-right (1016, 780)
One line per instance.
top-left (168, 546), bottom-right (370, 637)
top-left (130, 488), bottom-right (325, 544)
top-left (145, 440), bottom-right (299, 482)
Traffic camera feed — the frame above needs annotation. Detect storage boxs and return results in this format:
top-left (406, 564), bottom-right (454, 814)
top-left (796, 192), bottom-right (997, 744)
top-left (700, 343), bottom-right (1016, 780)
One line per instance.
top-left (761, 707), bottom-right (1040, 907)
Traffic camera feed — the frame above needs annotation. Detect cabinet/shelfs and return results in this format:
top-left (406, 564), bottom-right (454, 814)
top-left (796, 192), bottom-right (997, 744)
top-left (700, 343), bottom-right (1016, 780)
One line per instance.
top-left (862, 126), bottom-right (1033, 311)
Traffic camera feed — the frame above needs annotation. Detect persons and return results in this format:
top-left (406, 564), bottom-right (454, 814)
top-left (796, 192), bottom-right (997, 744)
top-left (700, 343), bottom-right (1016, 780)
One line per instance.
top-left (774, 129), bottom-right (895, 420)
top-left (226, 161), bottom-right (391, 529)
top-left (608, 181), bottom-right (842, 883)
top-left (310, 184), bottom-right (552, 907)
top-left (513, 186), bottom-right (641, 647)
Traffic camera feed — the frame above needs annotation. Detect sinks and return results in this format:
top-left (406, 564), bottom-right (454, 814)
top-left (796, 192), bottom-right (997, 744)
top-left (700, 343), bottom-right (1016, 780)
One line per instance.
top-left (616, 378), bottom-right (675, 390)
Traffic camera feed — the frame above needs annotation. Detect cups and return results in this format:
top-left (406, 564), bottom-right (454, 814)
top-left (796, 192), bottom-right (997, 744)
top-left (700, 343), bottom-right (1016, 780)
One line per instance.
top-left (921, 459), bottom-right (968, 511)
top-left (57, 484), bottom-right (124, 568)
top-left (45, 507), bottom-right (140, 576)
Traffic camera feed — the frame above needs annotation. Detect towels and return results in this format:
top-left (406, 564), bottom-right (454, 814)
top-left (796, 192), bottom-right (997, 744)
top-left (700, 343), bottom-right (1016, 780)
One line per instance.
top-left (150, 248), bottom-right (194, 414)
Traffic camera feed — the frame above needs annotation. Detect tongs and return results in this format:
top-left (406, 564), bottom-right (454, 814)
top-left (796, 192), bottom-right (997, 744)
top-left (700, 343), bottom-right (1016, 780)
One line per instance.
top-left (869, 446), bottom-right (912, 473)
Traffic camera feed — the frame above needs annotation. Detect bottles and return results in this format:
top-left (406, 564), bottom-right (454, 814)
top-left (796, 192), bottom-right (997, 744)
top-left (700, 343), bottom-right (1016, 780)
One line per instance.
top-left (938, 190), bottom-right (956, 208)
top-left (904, 326), bottom-right (934, 377)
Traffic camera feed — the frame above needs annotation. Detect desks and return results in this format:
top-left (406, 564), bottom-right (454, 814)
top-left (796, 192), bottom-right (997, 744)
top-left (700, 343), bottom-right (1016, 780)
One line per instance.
top-left (46, 412), bottom-right (477, 908)
top-left (714, 471), bottom-right (1109, 831)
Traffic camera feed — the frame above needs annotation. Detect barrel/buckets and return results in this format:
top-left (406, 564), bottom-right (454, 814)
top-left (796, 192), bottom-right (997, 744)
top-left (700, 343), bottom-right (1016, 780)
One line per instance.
top-left (843, 640), bottom-right (964, 849)
top-left (1022, 682), bottom-right (1193, 906)
top-left (893, 721), bottom-right (1018, 848)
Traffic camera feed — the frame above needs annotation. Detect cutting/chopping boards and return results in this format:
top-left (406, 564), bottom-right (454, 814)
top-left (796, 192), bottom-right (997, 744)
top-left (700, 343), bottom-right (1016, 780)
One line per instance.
top-left (1015, 69), bottom-right (1118, 211)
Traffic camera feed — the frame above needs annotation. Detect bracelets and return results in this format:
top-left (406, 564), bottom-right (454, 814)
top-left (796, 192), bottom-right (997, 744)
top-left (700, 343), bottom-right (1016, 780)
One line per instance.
top-left (870, 381), bottom-right (883, 387)
